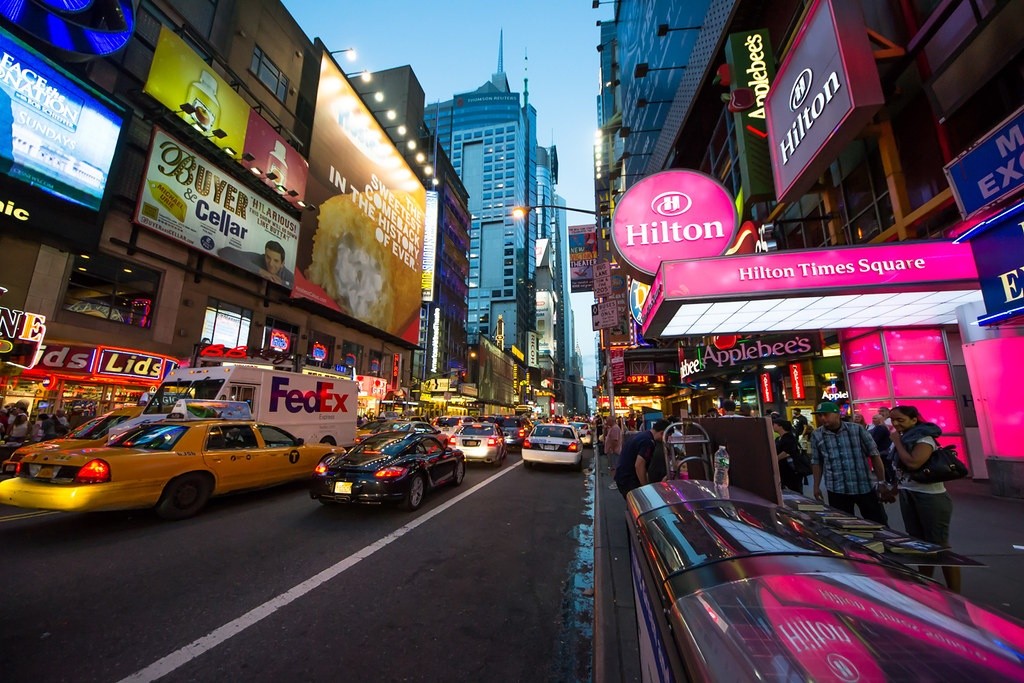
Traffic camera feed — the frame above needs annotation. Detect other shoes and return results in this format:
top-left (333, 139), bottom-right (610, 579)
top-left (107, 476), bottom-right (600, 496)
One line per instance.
top-left (608, 483), bottom-right (618, 489)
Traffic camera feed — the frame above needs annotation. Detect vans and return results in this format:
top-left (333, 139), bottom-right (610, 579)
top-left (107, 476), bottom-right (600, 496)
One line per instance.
top-left (109, 365), bottom-right (359, 452)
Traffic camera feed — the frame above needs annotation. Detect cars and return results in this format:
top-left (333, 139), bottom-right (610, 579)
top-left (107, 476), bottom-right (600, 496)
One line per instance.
top-left (306, 430), bottom-right (466, 513)
top-left (353, 413), bottom-right (533, 468)
top-left (0, 401), bottom-right (347, 525)
top-left (521, 421), bottom-right (592, 472)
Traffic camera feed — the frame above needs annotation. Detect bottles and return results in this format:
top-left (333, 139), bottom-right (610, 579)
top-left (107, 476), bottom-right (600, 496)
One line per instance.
top-left (714, 445), bottom-right (729, 489)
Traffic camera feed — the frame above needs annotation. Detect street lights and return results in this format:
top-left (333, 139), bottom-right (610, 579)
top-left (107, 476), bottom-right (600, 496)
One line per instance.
top-left (511, 193), bottom-right (616, 417)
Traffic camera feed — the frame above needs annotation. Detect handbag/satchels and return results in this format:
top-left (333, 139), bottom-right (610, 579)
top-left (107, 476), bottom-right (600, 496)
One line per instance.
top-left (798, 450), bottom-right (812, 477)
top-left (55, 425), bottom-right (68, 434)
top-left (911, 437), bottom-right (968, 484)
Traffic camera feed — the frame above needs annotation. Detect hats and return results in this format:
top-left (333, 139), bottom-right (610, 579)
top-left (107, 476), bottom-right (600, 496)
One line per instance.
top-left (810, 402), bottom-right (840, 414)
top-left (17, 399), bottom-right (29, 409)
top-left (719, 400), bottom-right (734, 408)
top-left (57, 409), bottom-right (66, 417)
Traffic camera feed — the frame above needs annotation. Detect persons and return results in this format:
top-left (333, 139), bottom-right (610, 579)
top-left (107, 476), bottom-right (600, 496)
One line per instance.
top-left (233, 241), bottom-right (294, 289)
top-left (358, 415), bottom-right (368, 426)
top-left (596, 403), bottom-right (962, 594)
top-left (0, 400), bottom-right (72, 449)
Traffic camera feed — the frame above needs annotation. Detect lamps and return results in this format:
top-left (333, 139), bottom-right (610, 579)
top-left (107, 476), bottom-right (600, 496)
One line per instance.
top-left (191, 122), bottom-right (208, 133)
top-left (206, 128), bottom-right (227, 141)
top-left (592, 0), bottom-right (621, 54)
top-left (234, 152), bottom-right (256, 162)
top-left (299, 204), bottom-right (318, 212)
top-left (637, 94), bottom-right (675, 109)
top-left (617, 123), bottom-right (664, 139)
top-left (260, 172), bottom-right (278, 181)
top-left (222, 146), bottom-right (237, 156)
top-left (272, 185), bottom-right (288, 193)
top-left (280, 190), bottom-right (299, 199)
top-left (330, 47), bottom-right (441, 186)
top-left (248, 167), bottom-right (263, 175)
top-left (630, 60), bottom-right (688, 79)
top-left (296, 200), bottom-right (306, 208)
top-left (173, 102), bottom-right (198, 117)
top-left (655, 22), bottom-right (703, 37)
top-left (600, 150), bottom-right (653, 214)
